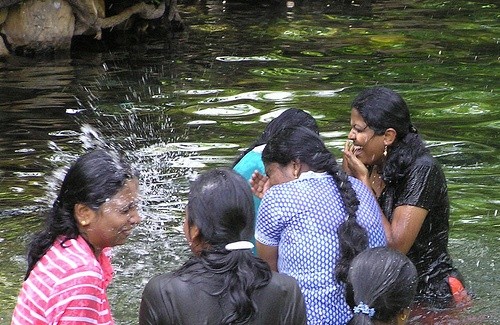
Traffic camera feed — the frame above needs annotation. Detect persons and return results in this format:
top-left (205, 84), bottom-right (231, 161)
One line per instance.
top-left (139, 168), bottom-right (307, 325)
top-left (342, 86), bottom-right (465, 312)
top-left (249, 126), bottom-right (389, 325)
top-left (345, 248), bottom-right (418, 325)
top-left (11, 150), bottom-right (142, 324)
top-left (232, 107), bottom-right (320, 256)
